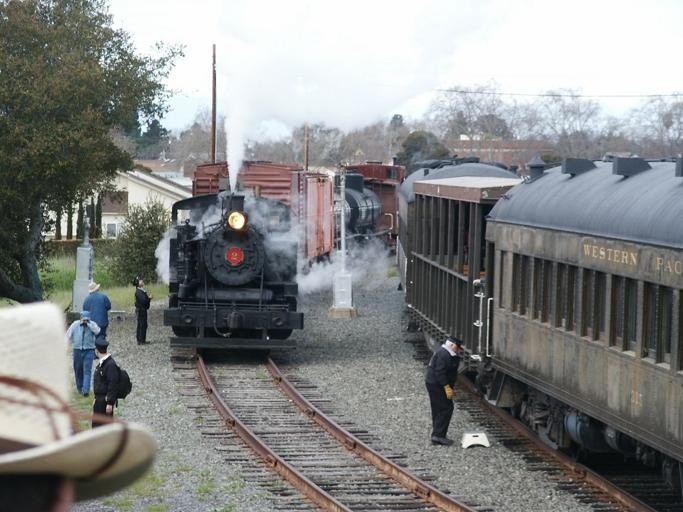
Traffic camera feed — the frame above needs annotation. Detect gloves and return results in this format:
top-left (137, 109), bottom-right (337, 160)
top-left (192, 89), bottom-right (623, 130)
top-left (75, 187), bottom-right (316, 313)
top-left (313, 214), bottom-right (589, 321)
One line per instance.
top-left (444, 384), bottom-right (456, 400)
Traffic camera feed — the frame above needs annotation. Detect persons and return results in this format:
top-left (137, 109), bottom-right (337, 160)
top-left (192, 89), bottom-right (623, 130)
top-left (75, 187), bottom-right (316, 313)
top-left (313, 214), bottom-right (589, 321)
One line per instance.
top-left (426, 336), bottom-right (463, 445)
top-left (92, 340), bottom-right (119, 428)
top-left (132, 276), bottom-right (152, 345)
top-left (66, 312), bottom-right (101, 397)
top-left (84, 282), bottom-right (111, 359)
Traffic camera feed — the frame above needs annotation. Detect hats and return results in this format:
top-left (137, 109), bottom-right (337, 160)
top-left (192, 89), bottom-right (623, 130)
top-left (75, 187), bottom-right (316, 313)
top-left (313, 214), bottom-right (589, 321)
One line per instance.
top-left (88, 282), bottom-right (101, 293)
top-left (133, 275), bottom-right (139, 285)
top-left (79, 310), bottom-right (90, 321)
top-left (447, 336), bottom-right (463, 348)
top-left (95, 339), bottom-right (109, 350)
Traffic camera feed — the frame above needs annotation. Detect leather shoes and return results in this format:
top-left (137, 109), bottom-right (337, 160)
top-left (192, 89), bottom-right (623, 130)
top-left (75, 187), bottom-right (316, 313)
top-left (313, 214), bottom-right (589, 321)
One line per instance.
top-left (138, 342), bottom-right (150, 345)
top-left (432, 436), bottom-right (450, 445)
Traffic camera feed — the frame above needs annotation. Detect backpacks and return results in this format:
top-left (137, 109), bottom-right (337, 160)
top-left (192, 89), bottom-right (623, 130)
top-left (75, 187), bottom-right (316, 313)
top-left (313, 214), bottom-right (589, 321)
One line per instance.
top-left (99, 363), bottom-right (132, 407)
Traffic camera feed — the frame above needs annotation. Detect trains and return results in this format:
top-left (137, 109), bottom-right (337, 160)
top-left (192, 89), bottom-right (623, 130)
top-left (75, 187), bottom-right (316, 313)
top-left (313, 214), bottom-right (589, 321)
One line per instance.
top-left (391, 151), bottom-right (682, 477)
top-left (161, 154), bottom-right (404, 355)
